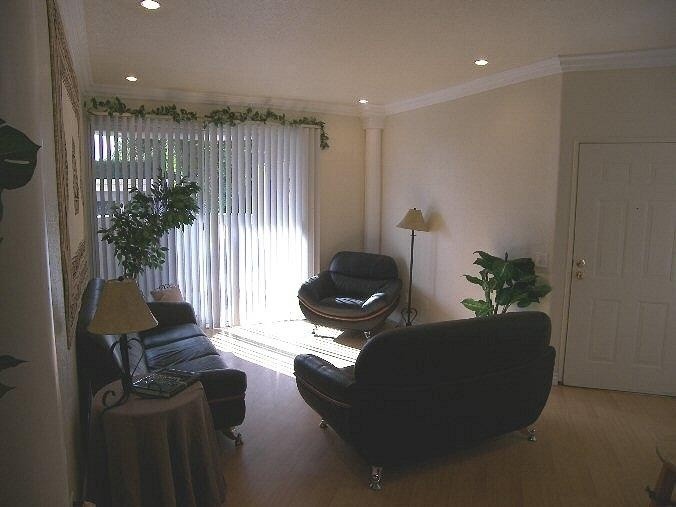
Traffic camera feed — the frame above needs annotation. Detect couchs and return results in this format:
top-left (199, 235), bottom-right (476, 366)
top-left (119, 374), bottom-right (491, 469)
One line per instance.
top-left (293, 310), bottom-right (556, 490)
top-left (76, 277), bottom-right (247, 447)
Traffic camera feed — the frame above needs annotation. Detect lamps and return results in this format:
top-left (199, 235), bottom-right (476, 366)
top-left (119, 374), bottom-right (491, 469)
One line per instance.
top-left (88, 277), bottom-right (162, 410)
top-left (395, 208), bottom-right (430, 326)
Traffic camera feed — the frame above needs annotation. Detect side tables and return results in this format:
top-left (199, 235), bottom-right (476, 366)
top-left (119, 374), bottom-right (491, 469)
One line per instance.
top-left (85, 372), bottom-right (228, 507)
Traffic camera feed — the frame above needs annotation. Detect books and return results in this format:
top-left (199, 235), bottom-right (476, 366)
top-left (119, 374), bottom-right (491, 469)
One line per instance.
top-left (130, 367), bottom-right (198, 399)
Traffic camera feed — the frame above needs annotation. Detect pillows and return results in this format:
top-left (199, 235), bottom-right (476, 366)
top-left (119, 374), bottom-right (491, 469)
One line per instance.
top-left (150, 284), bottom-right (184, 301)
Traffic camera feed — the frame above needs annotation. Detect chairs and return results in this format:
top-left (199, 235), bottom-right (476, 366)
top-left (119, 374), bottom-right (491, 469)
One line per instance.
top-left (297, 251), bottom-right (401, 343)
